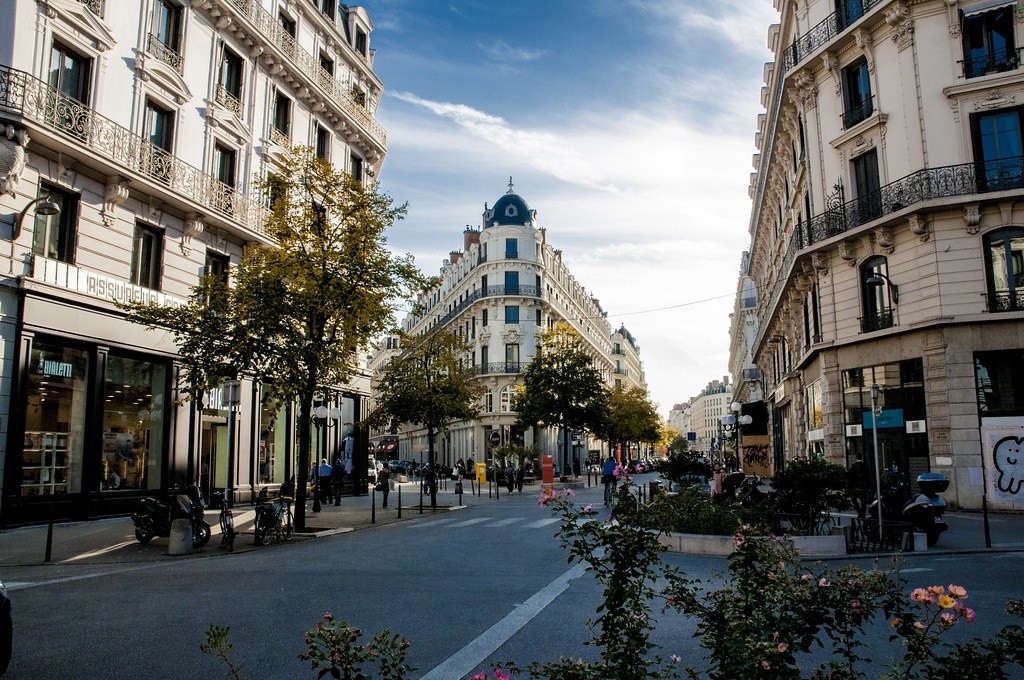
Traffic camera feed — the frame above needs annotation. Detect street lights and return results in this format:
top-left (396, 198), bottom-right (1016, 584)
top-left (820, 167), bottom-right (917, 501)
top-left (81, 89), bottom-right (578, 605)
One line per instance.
top-left (870, 382), bottom-right (886, 543)
top-left (298, 406), bottom-right (341, 511)
top-left (367, 454), bottom-right (455, 483)
top-left (572, 435), bottom-right (586, 468)
top-left (718, 401), bottom-right (753, 473)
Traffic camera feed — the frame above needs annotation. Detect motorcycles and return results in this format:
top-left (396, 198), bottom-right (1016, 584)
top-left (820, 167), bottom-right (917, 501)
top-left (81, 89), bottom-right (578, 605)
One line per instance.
top-left (131, 482), bottom-right (211, 549)
top-left (739, 472), bottom-right (768, 508)
top-left (864, 462), bottom-right (950, 547)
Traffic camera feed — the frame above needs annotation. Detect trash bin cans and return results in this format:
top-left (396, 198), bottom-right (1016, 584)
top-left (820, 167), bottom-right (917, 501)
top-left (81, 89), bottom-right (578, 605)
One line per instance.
top-left (649, 480), bottom-right (665, 502)
top-left (454, 482), bottom-right (464, 494)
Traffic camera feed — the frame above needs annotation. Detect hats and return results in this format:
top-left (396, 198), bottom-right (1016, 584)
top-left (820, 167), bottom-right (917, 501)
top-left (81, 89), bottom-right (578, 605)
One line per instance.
top-left (426, 463), bottom-right (429, 466)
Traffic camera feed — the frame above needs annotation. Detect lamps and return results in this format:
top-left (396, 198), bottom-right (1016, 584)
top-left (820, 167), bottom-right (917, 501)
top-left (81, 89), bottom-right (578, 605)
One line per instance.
top-left (867, 272), bottom-right (897, 306)
top-left (12, 195), bottom-right (60, 240)
top-left (769, 333), bottom-right (789, 343)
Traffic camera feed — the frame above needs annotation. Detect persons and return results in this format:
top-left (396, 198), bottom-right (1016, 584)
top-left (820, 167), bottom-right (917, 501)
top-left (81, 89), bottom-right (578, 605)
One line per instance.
top-left (313, 458), bottom-right (360, 506)
top-left (505, 461), bottom-right (515, 495)
top-left (377, 463), bottom-right (392, 508)
top-left (109, 468), bottom-right (120, 489)
top-left (423, 457), bottom-right (474, 496)
top-left (514, 463), bottom-right (525, 493)
top-left (602, 456), bottom-right (617, 505)
top-left (574, 456), bottom-right (629, 478)
top-left (710, 453), bottom-right (737, 498)
top-left (848, 453), bottom-right (868, 519)
top-left (412, 459), bottom-right (416, 477)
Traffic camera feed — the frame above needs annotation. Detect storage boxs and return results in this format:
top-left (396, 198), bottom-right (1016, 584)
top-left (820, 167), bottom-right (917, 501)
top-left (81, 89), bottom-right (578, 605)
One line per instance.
top-left (916, 473), bottom-right (951, 493)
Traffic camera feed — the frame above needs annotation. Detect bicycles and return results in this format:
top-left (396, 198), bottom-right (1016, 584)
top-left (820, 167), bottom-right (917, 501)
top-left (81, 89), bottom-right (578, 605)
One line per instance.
top-left (604, 478), bottom-right (621, 508)
top-left (253, 485), bottom-right (296, 547)
top-left (425, 479), bottom-right (438, 496)
top-left (213, 488), bottom-right (239, 551)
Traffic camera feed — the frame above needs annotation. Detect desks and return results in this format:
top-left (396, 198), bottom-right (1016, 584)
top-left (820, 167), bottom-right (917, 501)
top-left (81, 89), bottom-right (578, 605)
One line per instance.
top-left (820, 511), bottom-right (870, 548)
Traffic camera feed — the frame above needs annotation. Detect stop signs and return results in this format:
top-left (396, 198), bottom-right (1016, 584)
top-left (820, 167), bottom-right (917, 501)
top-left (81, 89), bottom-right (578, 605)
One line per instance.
top-left (491, 432), bottom-right (500, 448)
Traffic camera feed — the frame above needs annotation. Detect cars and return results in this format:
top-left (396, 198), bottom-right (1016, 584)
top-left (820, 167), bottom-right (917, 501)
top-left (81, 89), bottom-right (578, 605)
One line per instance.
top-left (670, 475), bottom-right (711, 498)
top-left (627, 456), bottom-right (671, 474)
top-left (0, 580), bottom-right (14, 680)
top-left (697, 455), bottom-right (717, 465)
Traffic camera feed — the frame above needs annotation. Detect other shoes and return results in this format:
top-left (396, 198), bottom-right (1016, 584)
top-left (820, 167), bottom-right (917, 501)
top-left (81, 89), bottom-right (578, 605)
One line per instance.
top-left (604, 502), bottom-right (607, 505)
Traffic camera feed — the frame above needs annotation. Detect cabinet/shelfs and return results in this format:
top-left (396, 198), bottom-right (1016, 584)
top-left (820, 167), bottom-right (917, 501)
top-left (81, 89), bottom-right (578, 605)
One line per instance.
top-left (104, 428), bottom-right (151, 480)
top-left (21, 431), bottom-right (73, 494)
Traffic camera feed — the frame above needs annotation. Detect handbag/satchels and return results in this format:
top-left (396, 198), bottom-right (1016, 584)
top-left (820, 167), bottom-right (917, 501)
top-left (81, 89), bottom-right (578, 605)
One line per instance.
top-left (375, 471), bottom-right (383, 491)
top-left (601, 477), bottom-right (611, 483)
top-left (505, 476), bottom-right (509, 483)
